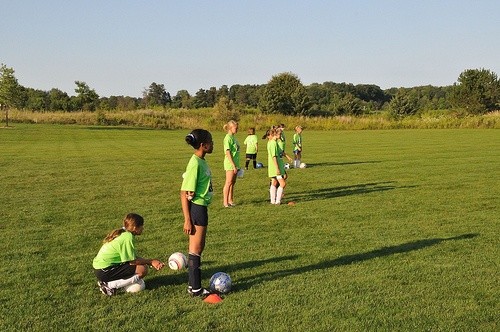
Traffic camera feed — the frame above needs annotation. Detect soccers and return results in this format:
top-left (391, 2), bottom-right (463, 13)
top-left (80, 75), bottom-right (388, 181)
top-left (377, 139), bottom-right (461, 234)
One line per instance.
top-left (123, 278), bottom-right (146, 292)
top-left (257, 162), bottom-right (263, 167)
top-left (168, 250), bottom-right (186, 270)
top-left (285, 162), bottom-right (293, 169)
top-left (210, 272), bottom-right (232, 294)
top-left (299, 162), bottom-right (307, 169)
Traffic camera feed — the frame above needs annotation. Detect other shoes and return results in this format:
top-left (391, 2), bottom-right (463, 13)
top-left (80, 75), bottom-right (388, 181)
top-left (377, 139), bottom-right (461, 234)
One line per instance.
top-left (224, 206), bottom-right (229, 208)
top-left (230, 203), bottom-right (236, 206)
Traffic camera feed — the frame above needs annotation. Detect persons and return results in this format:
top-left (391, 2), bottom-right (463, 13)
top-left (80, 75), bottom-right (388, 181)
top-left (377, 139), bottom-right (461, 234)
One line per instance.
top-left (92, 213), bottom-right (166, 296)
top-left (244, 124), bottom-right (302, 204)
top-left (223, 119), bottom-right (239, 207)
top-left (179, 129), bottom-right (213, 296)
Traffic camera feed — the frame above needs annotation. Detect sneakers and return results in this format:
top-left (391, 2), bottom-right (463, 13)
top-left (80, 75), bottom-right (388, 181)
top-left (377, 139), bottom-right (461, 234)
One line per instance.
top-left (98, 281), bottom-right (113, 296)
top-left (188, 286), bottom-right (212, 298)
